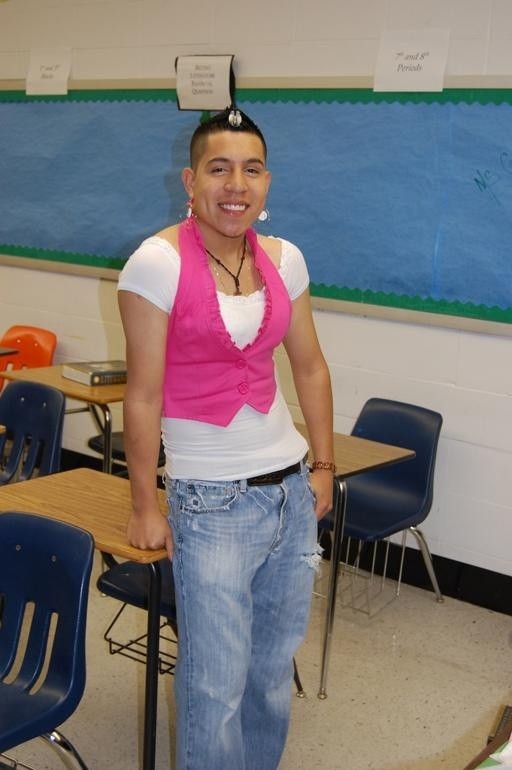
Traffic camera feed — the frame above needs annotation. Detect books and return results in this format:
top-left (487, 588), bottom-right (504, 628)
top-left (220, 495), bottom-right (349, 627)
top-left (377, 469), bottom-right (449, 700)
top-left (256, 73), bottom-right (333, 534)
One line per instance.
top-left (59, 360), bottom-right (129, 386)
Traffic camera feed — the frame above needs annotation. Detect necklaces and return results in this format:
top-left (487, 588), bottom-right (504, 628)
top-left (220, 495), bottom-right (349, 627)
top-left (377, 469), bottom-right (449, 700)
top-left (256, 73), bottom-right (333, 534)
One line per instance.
top-left (201, 233), bottom-right (253, 296)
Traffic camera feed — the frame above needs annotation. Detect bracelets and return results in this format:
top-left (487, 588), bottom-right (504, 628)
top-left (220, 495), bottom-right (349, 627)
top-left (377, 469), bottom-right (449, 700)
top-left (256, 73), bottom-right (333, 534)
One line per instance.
top-left (312, 461), bottom-right (337, 474)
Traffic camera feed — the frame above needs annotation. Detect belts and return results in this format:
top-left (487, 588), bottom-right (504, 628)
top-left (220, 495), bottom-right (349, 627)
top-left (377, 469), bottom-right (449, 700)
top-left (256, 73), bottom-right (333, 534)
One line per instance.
top-left (228, 451), bottom-right (311, 487)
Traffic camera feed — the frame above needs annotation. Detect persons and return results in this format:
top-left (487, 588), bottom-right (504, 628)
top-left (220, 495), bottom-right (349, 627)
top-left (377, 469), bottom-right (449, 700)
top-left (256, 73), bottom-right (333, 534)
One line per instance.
top-left (115, 108), bottom-right (338, 769)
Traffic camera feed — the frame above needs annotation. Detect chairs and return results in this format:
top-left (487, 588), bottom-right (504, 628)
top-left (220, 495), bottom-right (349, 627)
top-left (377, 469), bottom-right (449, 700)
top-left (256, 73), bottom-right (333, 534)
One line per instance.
top-left (0, 323), bottom-right (58, 394)
top-left (314, 395), bottom-right (446, 701)
top-left (1, 510), bottom-right (96, 755)
top-left (0, 379), bottom-right (69, 488)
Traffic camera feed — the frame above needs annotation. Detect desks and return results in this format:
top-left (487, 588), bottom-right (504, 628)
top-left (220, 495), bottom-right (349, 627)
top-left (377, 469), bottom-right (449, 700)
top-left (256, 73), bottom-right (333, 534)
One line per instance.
top-left (0, 357), bottom-right (137, 475)
top-left (0, 463), bottom-right (175, 770)
top-left (288, 423), bottom-right (418, 700)
